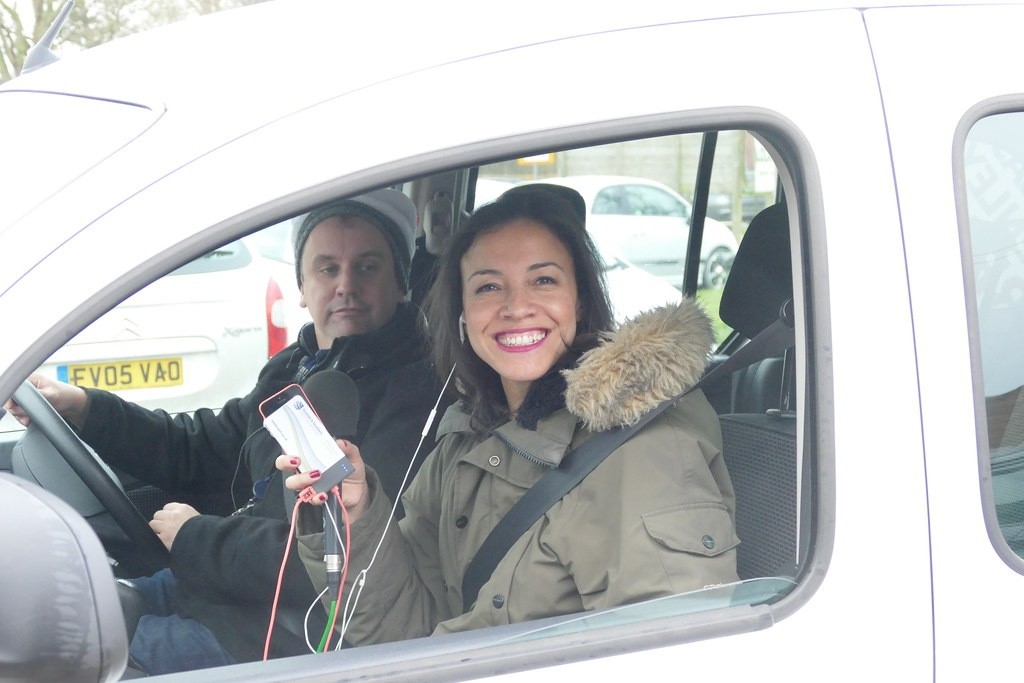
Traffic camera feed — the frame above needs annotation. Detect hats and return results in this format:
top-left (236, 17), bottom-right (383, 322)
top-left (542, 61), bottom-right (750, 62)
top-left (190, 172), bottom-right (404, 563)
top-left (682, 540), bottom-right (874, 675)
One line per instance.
top-left (293, 199), bottom-right (410, 295)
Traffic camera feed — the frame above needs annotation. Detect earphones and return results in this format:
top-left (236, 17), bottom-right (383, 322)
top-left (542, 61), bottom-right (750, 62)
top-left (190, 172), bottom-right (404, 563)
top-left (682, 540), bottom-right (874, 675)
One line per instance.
top-left (458, 310), bottom-right (466, 341)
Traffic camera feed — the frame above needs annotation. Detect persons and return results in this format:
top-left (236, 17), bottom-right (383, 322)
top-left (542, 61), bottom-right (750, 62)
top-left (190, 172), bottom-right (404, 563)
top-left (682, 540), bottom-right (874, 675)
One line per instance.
top-left (276, 197), bottom-right (743, 646)
top-left (3, 189), bottom-right (447, 676)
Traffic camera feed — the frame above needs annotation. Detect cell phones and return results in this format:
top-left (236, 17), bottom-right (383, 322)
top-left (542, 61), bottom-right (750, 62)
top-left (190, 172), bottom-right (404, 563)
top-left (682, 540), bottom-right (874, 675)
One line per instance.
top-left (258, 384), bottom-right (327, 475)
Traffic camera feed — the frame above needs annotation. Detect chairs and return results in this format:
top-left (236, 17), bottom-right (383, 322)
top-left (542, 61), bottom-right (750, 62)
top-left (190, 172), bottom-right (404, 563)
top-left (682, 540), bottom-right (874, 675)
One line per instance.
top-left (713, 204), bottom-right (804, 583)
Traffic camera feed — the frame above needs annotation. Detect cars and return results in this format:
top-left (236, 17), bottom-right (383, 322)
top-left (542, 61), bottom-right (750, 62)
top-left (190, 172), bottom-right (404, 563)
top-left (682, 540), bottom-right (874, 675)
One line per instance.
top-left (0, 0), bottom-right (1022, 683)
top-left (0, 174), bottom-right (755, 432)
